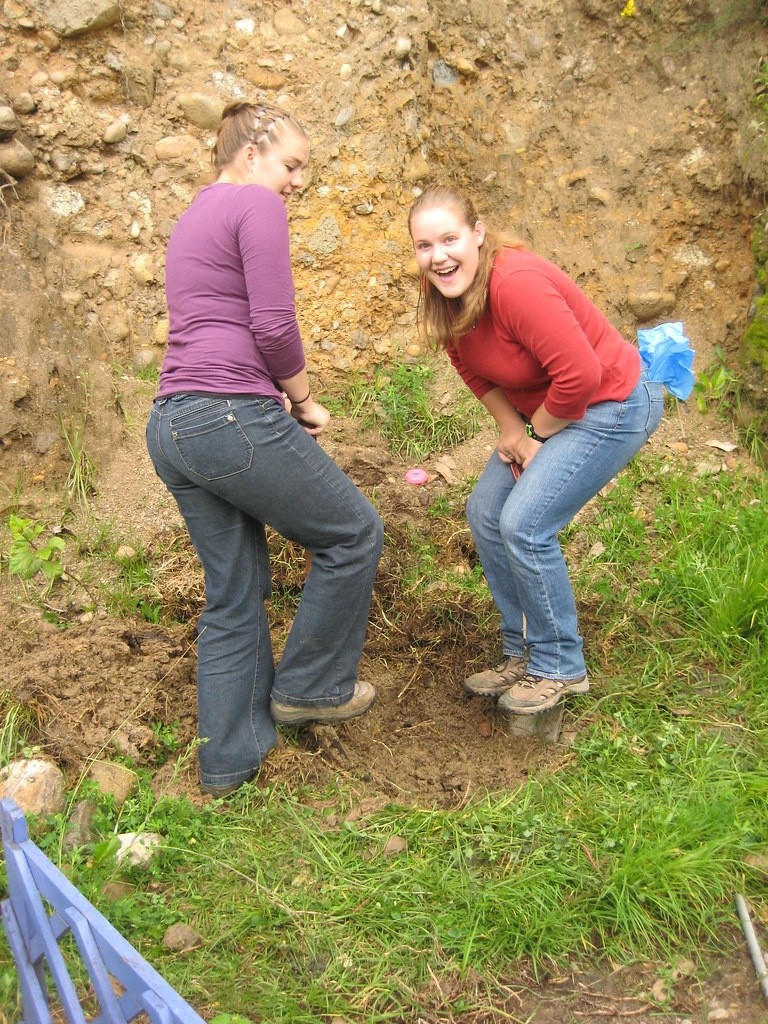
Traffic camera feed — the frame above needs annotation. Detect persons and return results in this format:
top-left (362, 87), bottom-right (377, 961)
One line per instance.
top-left (146, 100), bottom-right (384, 798)
top-left (408, 186), bottom-right (664, 713)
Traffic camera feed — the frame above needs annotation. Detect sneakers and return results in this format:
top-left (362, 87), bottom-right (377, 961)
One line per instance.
top-left (465, 655), bottom-right (530, 697)
top-left (199, 732), bottom-right (285, 798)
top-left (497, 673), bottom-right (590, 716)
top-left (270, 681), bottom-right (376, 724)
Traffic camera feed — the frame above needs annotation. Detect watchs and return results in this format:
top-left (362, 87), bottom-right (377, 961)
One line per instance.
top-left (525, 421), bottom-right (548, 443)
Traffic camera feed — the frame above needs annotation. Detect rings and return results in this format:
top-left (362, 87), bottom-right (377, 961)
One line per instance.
top-left (507, 454), bottom-right (511, 458)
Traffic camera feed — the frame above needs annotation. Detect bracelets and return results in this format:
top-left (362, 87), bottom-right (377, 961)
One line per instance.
top-left (289, 389), bottom-right (310, 404)
